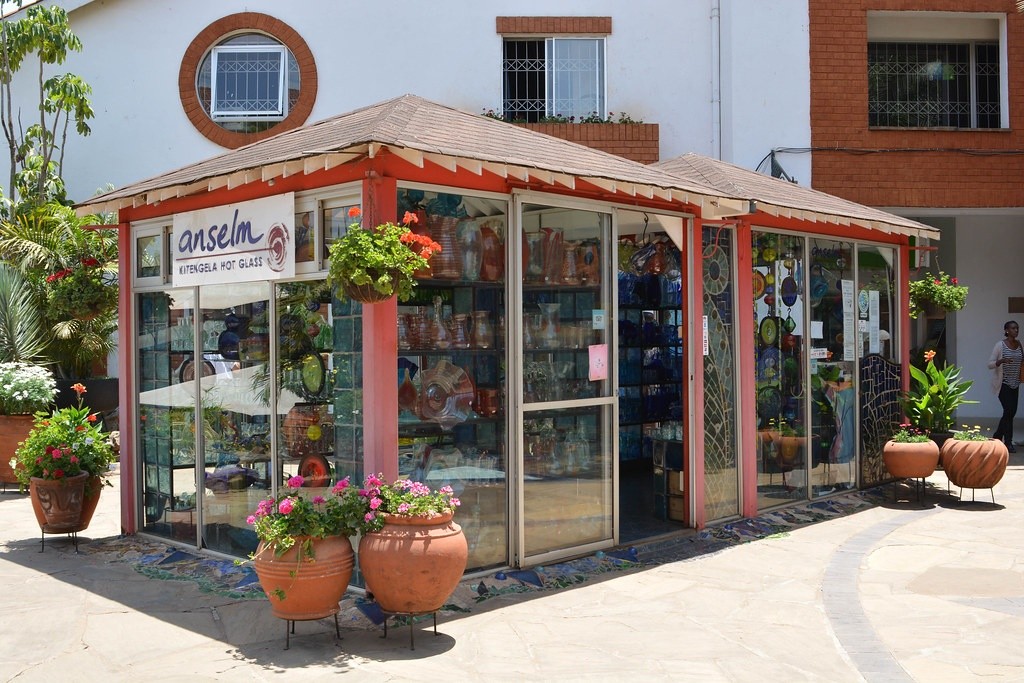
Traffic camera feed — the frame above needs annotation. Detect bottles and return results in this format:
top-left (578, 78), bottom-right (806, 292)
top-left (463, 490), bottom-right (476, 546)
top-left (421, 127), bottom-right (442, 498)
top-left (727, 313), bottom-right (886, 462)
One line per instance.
top-left (525, 416), bottom-right (590, 475)
top-left (449, 310), bottom-right (469, 346)
top-left (413, 296), bottom-right (452, 349)
top-left (476, 388), bottom-right (498, 418)
top-left (398, 314), bottom-right (414, 351)
top-left (470, 311), bottom-right (495, 347)
top-left (524, 313), bottom-right (542, 349)
top-left (406, 204), bottom-right (434, 279)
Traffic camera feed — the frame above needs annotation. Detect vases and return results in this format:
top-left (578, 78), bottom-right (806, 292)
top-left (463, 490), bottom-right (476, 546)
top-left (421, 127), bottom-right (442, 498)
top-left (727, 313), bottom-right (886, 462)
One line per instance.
top-left (357, 511), bottom-right (469, 616)
top-left (882, 439), bottom-right (939, 478)
top-left (929, 429), bottom-right (958, 470)
top-left (0, 415), bottom-right (36, 484)
top-left (254, 532), bottom-right (354, 620)
top-left (342, 270), bottom-right (398, 303)
top-left (939, 438), bottom-right (1010, 489)
top-left (30, 470), bottom-right (90, 529)
top-left (923, 300), bottom-right (945, 319)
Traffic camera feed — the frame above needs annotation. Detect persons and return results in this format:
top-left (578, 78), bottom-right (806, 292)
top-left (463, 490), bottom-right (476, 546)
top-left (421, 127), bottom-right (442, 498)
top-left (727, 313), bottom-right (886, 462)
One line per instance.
top-left (988, 320), bottom-right (1024, 453)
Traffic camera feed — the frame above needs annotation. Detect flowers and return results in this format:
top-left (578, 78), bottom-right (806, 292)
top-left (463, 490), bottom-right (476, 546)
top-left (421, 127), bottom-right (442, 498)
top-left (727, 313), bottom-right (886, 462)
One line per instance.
top-left (897, 349), bottom-right (980, 432)
top-left (954, 424), bottom-right (991, 441)
top-left (326, 206), bottom-right (442, 303)
top-left (891, 423), bottom-right (931, 443)
top-left (234, 473), bottom-right (461, 602)
top-left (908, 271), bottom-right (968, 320)
top-left (34, 443), bottom-right (80, 479)
top-left (70, 383), bottom-right (87, 399)
top-left (0, 361), bottom-right (60, 417)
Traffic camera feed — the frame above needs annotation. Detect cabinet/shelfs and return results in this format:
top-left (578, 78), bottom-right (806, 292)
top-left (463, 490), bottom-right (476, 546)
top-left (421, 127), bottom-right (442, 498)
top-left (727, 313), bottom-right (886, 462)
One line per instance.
top-left (138, 270), bottom-right (737, 538)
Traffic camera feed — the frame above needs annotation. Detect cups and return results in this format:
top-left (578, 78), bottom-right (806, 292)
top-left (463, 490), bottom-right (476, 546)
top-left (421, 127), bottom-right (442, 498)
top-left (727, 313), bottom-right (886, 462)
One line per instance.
top-left (562, 321), bottom-right (594, 348)
top-left (526, 232), bottom-right (546, 284)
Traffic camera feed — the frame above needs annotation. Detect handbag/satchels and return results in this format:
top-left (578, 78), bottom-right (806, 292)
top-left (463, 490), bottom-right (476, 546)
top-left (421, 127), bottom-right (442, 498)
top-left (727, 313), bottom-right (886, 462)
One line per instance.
top-left (1018, 340), bottom-right (1024, 384)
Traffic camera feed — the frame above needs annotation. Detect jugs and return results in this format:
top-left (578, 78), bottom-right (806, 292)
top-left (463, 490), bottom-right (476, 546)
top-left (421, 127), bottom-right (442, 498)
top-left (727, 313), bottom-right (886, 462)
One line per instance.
top-left (538, 303), bottom-right (562, 348)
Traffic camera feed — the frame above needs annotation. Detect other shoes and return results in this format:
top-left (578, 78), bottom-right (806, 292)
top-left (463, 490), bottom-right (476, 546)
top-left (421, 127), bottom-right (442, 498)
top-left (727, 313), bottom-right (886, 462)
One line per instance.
top-left (1004, 441), bottom-right (1016, 453)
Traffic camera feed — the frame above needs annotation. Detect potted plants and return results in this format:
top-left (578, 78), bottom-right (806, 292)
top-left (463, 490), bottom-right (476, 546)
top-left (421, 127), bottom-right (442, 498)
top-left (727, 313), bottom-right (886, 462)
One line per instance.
top-left (8, 404), bottom-right (115, 534)
